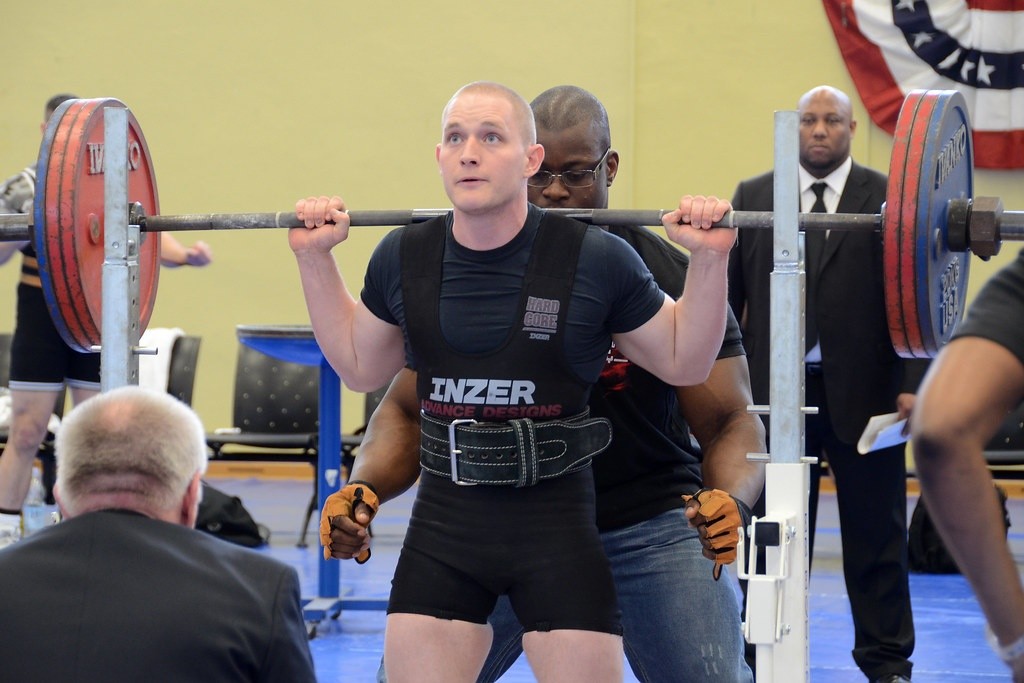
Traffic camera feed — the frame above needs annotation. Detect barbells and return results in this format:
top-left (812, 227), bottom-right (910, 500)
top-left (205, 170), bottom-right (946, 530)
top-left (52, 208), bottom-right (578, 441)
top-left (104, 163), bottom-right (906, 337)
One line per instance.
top-left (1, 96), bottom-right (1024, 361)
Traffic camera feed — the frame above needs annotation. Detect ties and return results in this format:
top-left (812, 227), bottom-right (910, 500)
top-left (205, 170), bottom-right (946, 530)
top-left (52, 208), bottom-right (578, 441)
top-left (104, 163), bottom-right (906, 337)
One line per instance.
top-left (805, 182), bottom-right (828, 278)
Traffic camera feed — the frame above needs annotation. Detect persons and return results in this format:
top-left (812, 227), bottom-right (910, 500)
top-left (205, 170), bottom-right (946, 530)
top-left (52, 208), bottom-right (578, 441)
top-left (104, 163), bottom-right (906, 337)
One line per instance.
top-left (719, 85), bottom-right (937, 683)
top-left (288, 80), bottom-right (738, 683)
top-left (0, 387), bottom-right (317, 683)
top-left (911, 247), bottom-right (1024, 683)
top-left (320, 86), bottom-right (766, 683)
top-left (0, 94), bottom-right (212, 553)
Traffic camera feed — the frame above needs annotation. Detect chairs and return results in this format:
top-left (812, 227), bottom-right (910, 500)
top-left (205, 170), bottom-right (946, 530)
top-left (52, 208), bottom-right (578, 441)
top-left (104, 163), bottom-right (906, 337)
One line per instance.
top-left (294, 377), bottom-right (390, 547)
top-left (202, 325), bottom-right (324, 548)
top-left (139, 336), bottom-right (200, 411)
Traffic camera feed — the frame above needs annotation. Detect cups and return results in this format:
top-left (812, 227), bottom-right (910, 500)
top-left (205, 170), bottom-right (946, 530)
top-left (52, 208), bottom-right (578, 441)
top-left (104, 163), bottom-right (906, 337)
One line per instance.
top-left (23, 507), bottom-right (61, 536)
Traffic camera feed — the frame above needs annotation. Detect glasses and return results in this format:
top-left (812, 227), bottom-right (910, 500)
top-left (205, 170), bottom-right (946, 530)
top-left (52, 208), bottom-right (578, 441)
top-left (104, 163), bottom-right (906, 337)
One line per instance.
top-left (527, 146), bottom-right (612, 188)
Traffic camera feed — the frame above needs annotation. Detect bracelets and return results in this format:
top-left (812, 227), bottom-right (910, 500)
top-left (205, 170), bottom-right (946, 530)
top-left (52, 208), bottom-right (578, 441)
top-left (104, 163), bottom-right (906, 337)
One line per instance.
top-left (988, 632), bottom-right (1024, 663)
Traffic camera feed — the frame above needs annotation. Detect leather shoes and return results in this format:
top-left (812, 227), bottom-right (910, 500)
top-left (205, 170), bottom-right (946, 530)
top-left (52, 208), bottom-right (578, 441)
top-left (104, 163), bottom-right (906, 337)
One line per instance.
top-left (877, 673), bottom-right (912, 683)
